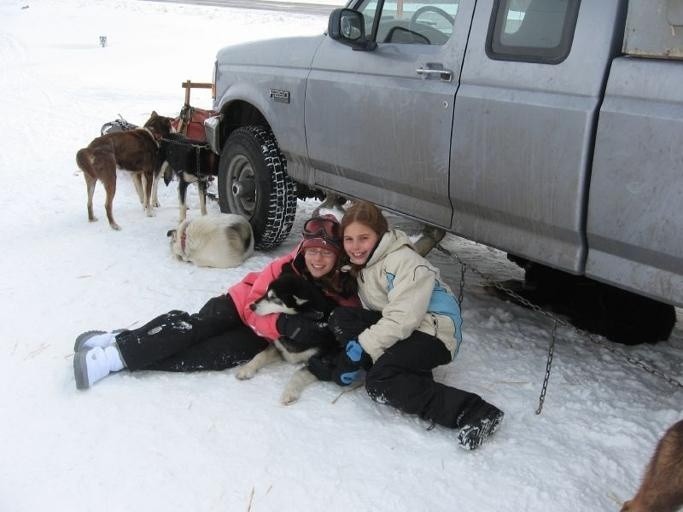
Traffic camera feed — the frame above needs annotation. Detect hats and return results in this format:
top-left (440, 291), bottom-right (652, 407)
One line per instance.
top-left (300, 214), bottom-right (343, 260)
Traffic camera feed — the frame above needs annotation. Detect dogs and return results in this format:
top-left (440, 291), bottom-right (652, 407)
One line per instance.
top-left (468, 250), bottom-right (677, 348)
top-left (152, 131), bottom-right (221, 223)
top-left (235, 261), bottom-right (384, 405)
top-left (165, 212), bottom-right (257, 270)
top-left (617, 418), bottom-right (683, 512)
top-left (75, 110), bottom-right (176, 231)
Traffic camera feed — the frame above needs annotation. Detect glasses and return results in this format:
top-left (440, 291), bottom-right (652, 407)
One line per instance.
top-left (302, 217), bottom-right (342, 251)
top-left (305, 246), bottom-right (334, 258)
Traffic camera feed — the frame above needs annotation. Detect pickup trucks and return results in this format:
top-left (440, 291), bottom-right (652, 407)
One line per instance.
top-left (200, 1), bottom-right (683, 311)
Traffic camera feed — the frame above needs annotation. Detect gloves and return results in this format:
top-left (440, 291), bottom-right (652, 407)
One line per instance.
top-left (331, 337), bottom-right (372, 386)
top-left (276, 312), bottom-right (318, 346)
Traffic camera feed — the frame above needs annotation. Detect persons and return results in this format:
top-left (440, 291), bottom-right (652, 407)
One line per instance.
top-left (71, 212), bottom-right (362, 391)
top-left (325, 198), bottom-right (506, 452)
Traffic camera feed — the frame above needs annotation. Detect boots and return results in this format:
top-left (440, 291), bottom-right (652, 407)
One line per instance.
top-left (73, 328), bottom-right (128, 351)
top-left (455, 394), bottom-right (505, 450)
top-left (73, 344), bottom-right (128, 389)
